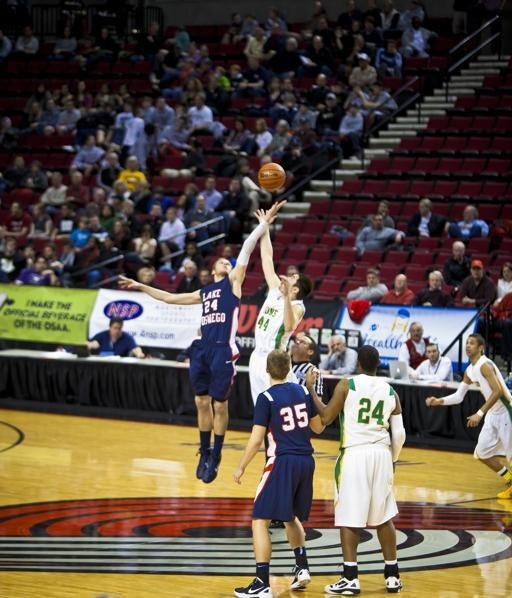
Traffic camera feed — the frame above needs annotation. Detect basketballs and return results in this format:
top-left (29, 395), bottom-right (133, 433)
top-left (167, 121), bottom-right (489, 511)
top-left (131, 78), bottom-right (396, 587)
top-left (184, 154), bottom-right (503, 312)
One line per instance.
top-left (258, 163), bottom-right (286, 190)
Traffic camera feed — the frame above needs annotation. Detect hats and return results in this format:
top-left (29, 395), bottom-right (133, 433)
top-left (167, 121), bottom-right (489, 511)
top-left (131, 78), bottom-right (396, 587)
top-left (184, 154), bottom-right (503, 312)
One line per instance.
top-left (470, 259), bottom-right (481, 269)
top-left (356, 52), bottom-right (369, 62)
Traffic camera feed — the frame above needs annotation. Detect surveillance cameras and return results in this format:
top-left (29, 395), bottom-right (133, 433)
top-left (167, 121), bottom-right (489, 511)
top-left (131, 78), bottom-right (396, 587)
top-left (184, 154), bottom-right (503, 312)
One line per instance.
top-left (0, 292), bottom-right (15, 313)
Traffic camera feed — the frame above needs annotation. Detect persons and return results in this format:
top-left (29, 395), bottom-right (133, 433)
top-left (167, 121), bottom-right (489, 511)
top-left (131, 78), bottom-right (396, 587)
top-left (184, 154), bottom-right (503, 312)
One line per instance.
top-left (249, 209), bottom-right (312, 407)
top-left (318, 335), bottom-right (358, 375)
top-left (452, 0), bottom-right (508, 61)
top-left (234, 350), bottom-right (327, 598)
top-left (341, 198), bottom-right (512, 365)
top-left (342, 240), bottom-right (512, 318)
top-left (426, 334), bottom-right (512, 499)
top-left (306, 345), bottom-right (405, 596)
top-left (270, 330), bottom-right (325, 530)
top-left (118, 199), bottom-right (289, 484)
top-left (85, 317), bottom-right (145, 358)
top-left (399, 322), bottom-right (434, 376)
top-left (2, 1), bottom-right (439, 295)
top-left (177, 339), bottom-right (201, 363)
top-left (410, 343), bottom-right (453, 381)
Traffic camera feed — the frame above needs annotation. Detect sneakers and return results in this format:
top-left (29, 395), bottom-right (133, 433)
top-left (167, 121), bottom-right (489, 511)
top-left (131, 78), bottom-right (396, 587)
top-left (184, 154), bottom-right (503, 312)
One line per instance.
top-left (498, 485), bottom-right (511, 499)
top-left (202, 453), bottom-right (221, 482)
top-left (324, 578), bottom-right (362, 596)
top-left (234, 583), bottom-right (272, 598)
top-left (197, 449), bottom-right (209, 479)
top-left (290, 569), bottom-right (313, 592)
top-left (387, 575), bottom-right (402, 593)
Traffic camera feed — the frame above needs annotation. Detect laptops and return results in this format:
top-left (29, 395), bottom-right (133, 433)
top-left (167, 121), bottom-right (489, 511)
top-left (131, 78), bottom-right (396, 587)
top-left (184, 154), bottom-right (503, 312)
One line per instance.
top-left (388, 360), bottom-right (422, 382)
top-left (72, 344), bottom-right (91, 358)
